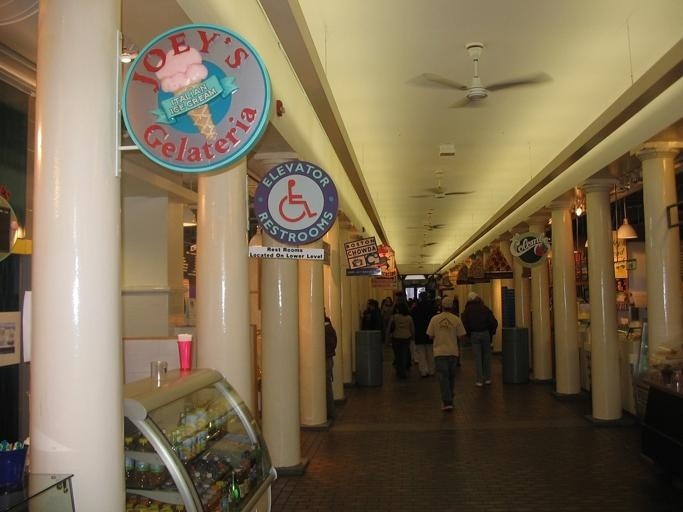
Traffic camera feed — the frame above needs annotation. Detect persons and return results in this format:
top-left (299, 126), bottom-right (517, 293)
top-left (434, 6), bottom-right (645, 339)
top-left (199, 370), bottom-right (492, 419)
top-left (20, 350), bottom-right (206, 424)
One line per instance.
top-left (321, 306), bottom-right (338, 423)
top-left (425, 296), bottom-right (468, 410)
top-left (359, 289), bottom-right (498, 387)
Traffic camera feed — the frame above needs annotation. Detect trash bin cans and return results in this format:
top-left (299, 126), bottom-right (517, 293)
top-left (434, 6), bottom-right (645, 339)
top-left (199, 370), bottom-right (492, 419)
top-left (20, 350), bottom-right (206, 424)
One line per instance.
top-left (355, 329), bottom-right (382, 387)
top-left (502, 328), bottom-right (530, 385)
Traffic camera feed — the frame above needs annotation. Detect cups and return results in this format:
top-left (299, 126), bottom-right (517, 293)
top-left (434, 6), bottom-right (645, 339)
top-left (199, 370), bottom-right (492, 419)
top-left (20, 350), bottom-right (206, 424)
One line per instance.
top-left (150, 360), bottom-right (168, 382)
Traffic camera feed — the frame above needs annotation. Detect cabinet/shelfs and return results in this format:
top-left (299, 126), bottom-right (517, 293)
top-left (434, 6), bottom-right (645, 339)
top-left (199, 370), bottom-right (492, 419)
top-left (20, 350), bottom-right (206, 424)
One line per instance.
top-left (123, 369), bottom-right (279, 512)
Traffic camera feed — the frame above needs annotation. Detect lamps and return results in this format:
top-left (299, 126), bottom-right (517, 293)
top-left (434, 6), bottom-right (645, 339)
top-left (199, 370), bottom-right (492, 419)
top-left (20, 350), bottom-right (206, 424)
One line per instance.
top-left (615, 195), bottom-right (640, 239)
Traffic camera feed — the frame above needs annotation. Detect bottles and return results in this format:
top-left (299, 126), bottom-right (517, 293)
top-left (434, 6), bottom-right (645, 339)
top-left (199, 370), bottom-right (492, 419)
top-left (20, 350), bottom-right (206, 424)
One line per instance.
top-left (125, 400), bottom-right (257, 512)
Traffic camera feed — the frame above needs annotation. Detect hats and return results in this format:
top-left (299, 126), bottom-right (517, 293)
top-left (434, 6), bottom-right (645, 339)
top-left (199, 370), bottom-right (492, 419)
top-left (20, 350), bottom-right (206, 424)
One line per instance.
top-left (442, 296), bottom-right (454, 308)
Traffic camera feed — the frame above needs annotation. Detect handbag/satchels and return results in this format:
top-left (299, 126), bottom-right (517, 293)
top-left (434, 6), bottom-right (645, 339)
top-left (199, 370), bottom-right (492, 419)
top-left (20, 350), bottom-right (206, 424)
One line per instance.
top-left (389, 314), bottom-right (396, 334)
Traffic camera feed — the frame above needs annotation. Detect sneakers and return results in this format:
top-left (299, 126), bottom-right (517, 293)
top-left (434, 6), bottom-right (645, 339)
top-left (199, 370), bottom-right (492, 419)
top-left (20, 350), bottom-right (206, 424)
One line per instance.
top-left (475, 378), bottom-right (492, 388)
top-left (440, 402), bottom-right (454, 411)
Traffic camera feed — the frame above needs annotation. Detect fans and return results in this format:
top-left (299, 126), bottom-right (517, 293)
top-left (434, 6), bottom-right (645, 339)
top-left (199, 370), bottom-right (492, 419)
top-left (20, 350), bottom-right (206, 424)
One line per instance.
top-left (404, 170), bottom-right (477, 259)
top-left (420, 42), bottom-right (554, 108)
